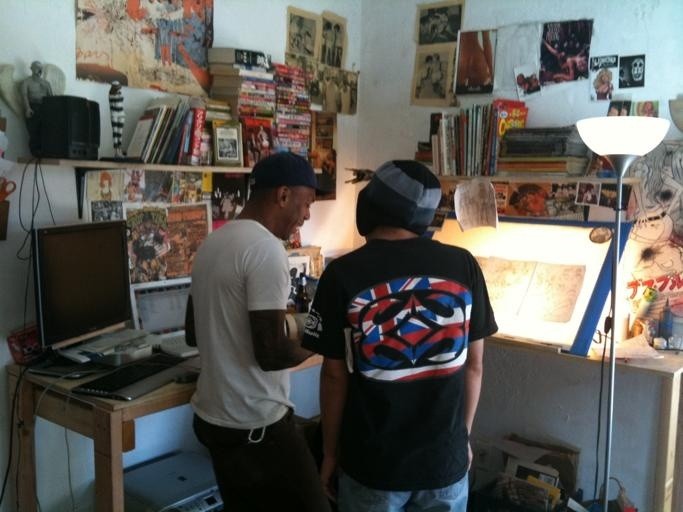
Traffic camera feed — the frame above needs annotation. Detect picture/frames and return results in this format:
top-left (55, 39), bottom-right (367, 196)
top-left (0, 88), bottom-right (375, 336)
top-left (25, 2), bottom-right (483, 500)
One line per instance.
top-left (285, 5), bottom-right (321, 61)
top-left (320, 11), bottom-right (348, 68)
top-left (410, 42), bottom-right (456, 107)
top-left (416, 0), bottom-right (466, 42)
top-left (212, 117), bottom-right (244, 166)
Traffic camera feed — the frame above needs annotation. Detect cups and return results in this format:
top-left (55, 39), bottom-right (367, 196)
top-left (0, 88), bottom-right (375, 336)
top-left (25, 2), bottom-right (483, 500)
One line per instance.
top-left (0, 177), bottom-right (16, 203)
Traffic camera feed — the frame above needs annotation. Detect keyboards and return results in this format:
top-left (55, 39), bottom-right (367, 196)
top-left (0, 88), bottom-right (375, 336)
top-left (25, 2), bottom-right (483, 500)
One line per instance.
top-left (71, 352), bottom-right (182, 402)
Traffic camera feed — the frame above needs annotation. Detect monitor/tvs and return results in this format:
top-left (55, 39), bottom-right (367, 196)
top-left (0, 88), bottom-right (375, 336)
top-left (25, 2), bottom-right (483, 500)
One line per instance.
top-left (31, 220), bottom-right (139, 364)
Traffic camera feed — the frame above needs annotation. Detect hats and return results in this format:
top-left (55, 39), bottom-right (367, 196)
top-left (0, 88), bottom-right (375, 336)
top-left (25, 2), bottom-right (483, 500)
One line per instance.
top-left (356, 160), bottom-right (442, 236)
top-left (249, 151), bottom-right (330, 195)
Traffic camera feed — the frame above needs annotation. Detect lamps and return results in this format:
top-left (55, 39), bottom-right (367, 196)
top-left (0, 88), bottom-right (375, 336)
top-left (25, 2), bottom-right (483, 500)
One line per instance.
top-left (575, 115), bottom-right (672, 510)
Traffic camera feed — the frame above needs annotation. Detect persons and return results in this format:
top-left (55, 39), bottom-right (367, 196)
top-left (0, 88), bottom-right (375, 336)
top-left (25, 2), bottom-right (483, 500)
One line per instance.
top-left (310, 157), bottom-right (497, 512)
top-left (99, 172), bottom-right (112, 200)
top-left (212, 187), bottom-right (240, 220)
top-left (593, 59), bottom-right (675, 341)
top-left (130, 214), bottom-right (169, 284)
top-left (184, 150), bottom-right (319, 512)
top-left (123, 184), bottom-right (140, 220)
top-left (289, 262), bottom-right (307, 307)
top-left (21, 61), bottom-right (53, 122)
top-left (124, 171), bottom-right (143, 194)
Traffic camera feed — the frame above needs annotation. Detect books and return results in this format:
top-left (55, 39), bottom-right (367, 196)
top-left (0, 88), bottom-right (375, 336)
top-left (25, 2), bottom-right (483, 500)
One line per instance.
top-left (502, 434), bottom-right (584, 508)
top-left (414, 96), bottom-right (587, 176)
top-left (132, 45), bottom-right (314, 171)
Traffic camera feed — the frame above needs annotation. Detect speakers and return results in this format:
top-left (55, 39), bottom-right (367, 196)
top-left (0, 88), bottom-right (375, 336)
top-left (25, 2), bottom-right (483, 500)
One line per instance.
top-left (27, 95), bottom-right (100, 160)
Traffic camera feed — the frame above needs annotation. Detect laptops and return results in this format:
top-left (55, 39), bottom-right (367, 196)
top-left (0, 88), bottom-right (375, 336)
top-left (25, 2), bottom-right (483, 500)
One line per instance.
top-left (130, 276), bottom-right (199, 361)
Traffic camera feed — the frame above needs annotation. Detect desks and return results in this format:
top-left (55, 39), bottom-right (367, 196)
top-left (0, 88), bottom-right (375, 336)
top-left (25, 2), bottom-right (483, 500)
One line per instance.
top-left (4, 348), bottom-right (324, 512)
top-left (487, 333), bottom-right (683, 511)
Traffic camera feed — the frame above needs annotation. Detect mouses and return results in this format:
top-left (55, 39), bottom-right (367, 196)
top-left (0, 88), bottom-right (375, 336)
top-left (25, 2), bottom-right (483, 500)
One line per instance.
top-left (176, 368), bottom-right (200, 383)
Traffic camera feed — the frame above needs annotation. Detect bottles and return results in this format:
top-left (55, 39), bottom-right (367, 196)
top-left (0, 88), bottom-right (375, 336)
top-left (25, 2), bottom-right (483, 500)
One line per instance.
top-left (294, 273), bottom-right (309, 313)
top-left (659, 297), bottom-right (674, 338)
